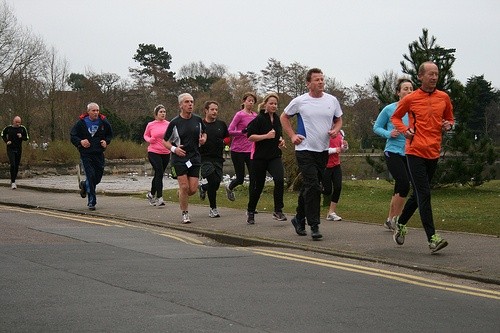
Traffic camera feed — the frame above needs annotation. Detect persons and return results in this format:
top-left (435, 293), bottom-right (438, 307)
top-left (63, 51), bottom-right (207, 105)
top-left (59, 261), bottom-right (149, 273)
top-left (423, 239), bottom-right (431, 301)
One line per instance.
top-left (322, 122), bottom-right (349, 221)
top-left (199, 101), bottom-right (231, 217)
top-left (280, 68), bottom-right (343, 237)
top-left (70, 103), bottom-right (113, 211)
top-left (1, 116), bottom-right (29, 189)
top-left (390, 61), bottom-right (454, 253)
top-left (226, 92), bottom-right (258, 213)
top-left (373, 78), bottom-right (413, 230)
top-left (247, 94), bottom-right (287, 224)
top-left (143, 105), bottom-right (170, 206)
top-left (162, 93), bottom-right (207, 223)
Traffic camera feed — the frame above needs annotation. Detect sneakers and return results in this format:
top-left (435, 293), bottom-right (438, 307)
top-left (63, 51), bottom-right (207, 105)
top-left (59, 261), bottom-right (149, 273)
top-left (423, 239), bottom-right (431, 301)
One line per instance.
top-left (245, 209), bottom-right (258, 224)
top-left (428, 234), bottom-right (448, 255)
top-left (272, 212), bottom-right (287, 221)
top-left (384, 218), bottom-right (392, 231)
top-left (79, 181), bottom-right (87, 198)
top-left (311, 230), bottom-right (323, 240)
top-left (209, 207), bottom-right (220, 218)
top-left (390, 217), bottom-right (397, 232)
top-left (291, 216), bottom-right (307, 236)
top-left (225, 183), bottom-right (235, 201)
top-left (177, 190), bottom-right (179, 198)
top-left (157, 197), bottom-right (165, 207)
top-left (146, 193), bottom-right (156, 206)
top-left (11, 183), bottom-right (17, 189)
top-left (327, 212), bottom-right (342, 221)
top-left (180, 210), bottom-right (192, 223)
top-left (393, 217), bottom-right (408, 247)
top-left (198, 184), bottom-right (206, 200)
top-left (89, 204), bottom-right (95, 210)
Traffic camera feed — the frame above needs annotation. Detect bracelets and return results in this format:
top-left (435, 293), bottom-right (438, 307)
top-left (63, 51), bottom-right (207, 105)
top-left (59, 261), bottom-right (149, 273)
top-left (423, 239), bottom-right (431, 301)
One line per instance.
top-left (171, 146), bottom-right (176, 152)
